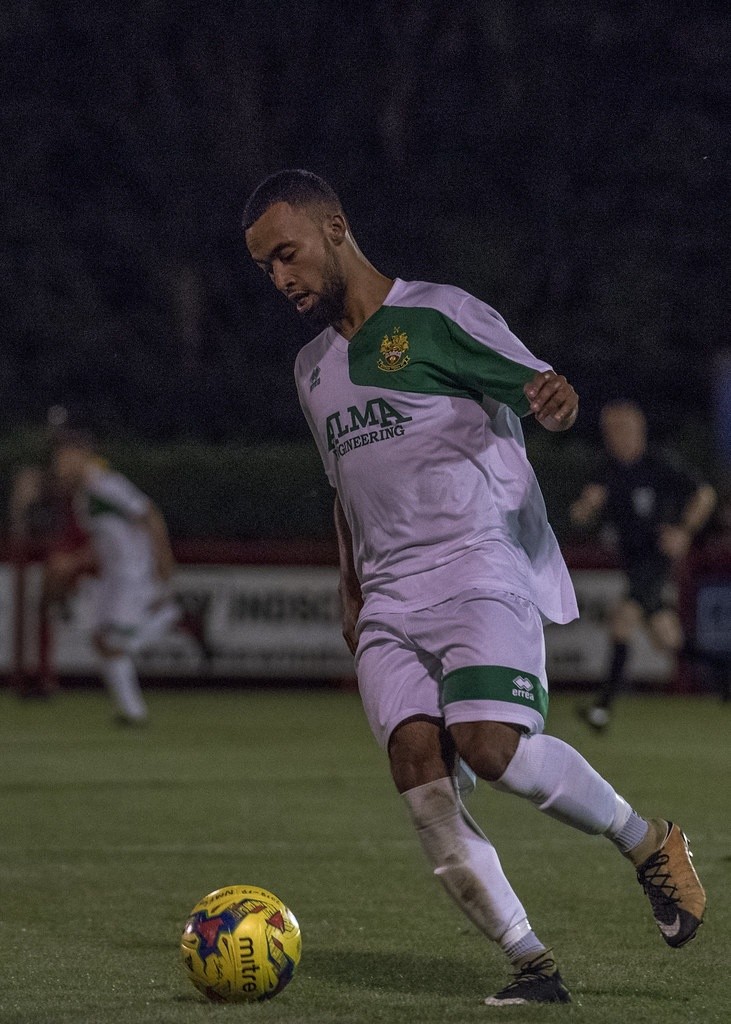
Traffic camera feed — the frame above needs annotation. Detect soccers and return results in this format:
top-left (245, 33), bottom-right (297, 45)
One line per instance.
top-left (180, 885), bottom-right (302, 1003)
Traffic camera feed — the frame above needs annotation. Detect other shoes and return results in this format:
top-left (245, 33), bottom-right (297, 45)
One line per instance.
top-left (574, 705), bottom-right (609, 734)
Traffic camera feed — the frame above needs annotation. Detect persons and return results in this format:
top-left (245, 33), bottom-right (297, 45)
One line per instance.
top-left (242, 170), bottom-right (707, 1005)
top-left (5, 398), bottom-right (717, 731)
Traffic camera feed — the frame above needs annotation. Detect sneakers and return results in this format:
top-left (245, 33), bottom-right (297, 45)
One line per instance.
top-left (635, 820), bottom-right (707, 949)
top-left (484, 948), bottom-right (576, 1006)
